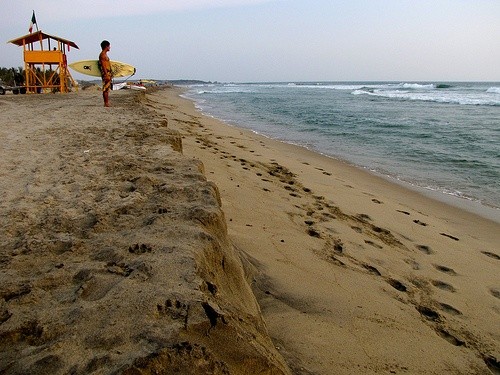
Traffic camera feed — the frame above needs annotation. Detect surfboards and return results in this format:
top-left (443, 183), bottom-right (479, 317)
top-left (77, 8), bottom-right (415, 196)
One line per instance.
top-left (68, 60), bottom-right (135, 78)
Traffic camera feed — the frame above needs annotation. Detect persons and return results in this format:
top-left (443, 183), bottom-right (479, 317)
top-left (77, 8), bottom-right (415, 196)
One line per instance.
top-left (99, 40), bottom-right (115, 108)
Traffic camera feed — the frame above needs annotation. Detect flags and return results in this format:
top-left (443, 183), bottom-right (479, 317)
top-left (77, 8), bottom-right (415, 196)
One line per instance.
top-left (29, 12), bottom-right (36, 34)
